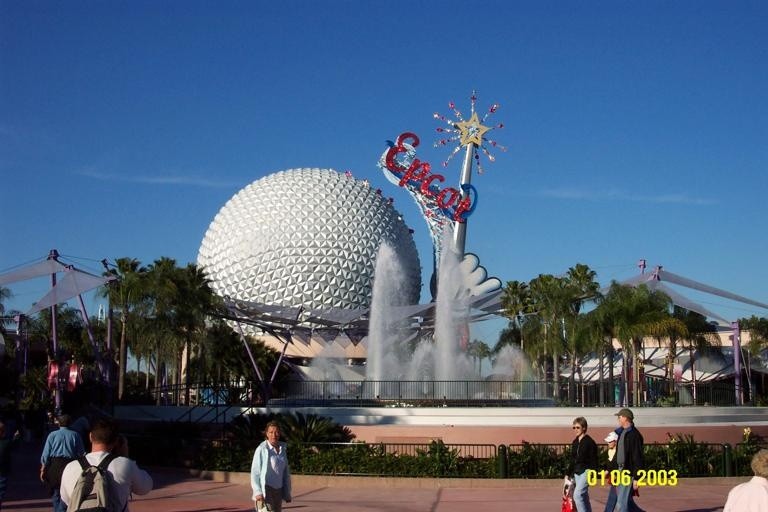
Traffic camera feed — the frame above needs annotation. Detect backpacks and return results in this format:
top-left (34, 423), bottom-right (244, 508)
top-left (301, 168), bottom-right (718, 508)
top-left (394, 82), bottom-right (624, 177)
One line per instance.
top-left (66, 452), bottom-right (127, 512)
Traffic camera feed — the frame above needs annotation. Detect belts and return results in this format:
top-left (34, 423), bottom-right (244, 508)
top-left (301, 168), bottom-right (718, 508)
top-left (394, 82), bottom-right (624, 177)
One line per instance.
top-left (617, 463), bottom-right (625, 467)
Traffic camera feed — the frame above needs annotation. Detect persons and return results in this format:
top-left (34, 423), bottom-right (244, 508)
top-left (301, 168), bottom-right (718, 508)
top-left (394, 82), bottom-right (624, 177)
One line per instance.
top-left (723, 448), bottom-right (768, 512)
top-left (0, 402), bottom-right (25, 508)
top-left (250, 421), bottom-right (292, 512)
top-left (564, 417), bottom-right (598, 512)
top-left (69, 413), bottom-right (92, 437)
top-left (604, 431), bottom-right (619, 512)
top-left (604, 408), bottom-right (645, 512)
top-left (40, 414), bottom-right (86, 512)
top-left (60, 420), bottom-right (153, 512)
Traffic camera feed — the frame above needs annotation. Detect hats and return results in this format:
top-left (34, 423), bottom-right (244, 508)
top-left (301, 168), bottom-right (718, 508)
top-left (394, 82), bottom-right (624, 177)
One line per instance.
top-left (604, 431), bottom-right (619, 442)
top-left (256, 498), bottom-right (272, 512)
top-left (614, 408), bottom-right (633, 419)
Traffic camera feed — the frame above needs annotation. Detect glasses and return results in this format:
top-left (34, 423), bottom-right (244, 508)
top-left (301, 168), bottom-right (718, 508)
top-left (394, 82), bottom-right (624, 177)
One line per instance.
top-left (573, 427), bottom-right (582, 429)
